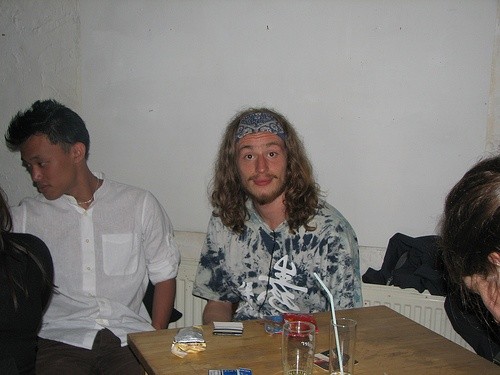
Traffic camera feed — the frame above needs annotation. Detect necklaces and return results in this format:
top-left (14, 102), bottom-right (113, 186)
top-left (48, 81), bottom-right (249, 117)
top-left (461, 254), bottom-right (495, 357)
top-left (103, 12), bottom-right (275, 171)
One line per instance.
top-left (78, 177), bottom-right (100, 205)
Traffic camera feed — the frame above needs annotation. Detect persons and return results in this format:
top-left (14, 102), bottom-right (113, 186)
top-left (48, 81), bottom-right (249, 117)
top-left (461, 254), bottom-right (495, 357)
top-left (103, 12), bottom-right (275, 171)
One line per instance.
top-left (192, 106), bottom-right (363, 325)
top-left (0, 98), bottom-right (181, 375)
top-left (0, 180), bottom-right (58, 375)
top-left (441, 156), bottom-right (500, 322)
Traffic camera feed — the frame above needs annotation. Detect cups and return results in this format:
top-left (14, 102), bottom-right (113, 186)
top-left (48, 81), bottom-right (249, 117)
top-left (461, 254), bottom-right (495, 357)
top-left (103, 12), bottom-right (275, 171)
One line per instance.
top-left (330, 318), bottom-right (358, 375)
top-left (282, 321), bottom-right (315, 375)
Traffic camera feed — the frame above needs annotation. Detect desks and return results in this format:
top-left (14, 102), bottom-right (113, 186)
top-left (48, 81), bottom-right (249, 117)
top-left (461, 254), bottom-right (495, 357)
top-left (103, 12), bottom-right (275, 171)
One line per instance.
top-left (127, 306), bottom-right (500, 374)
